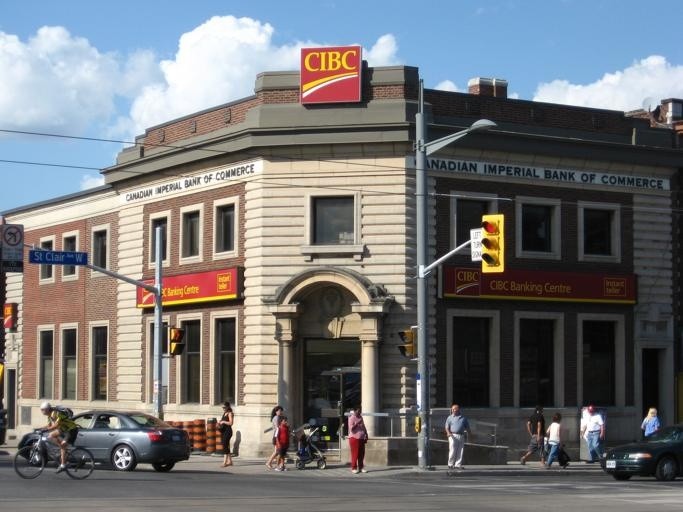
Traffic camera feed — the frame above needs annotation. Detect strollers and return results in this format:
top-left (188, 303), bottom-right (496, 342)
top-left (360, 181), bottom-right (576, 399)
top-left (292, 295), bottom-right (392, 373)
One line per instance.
top-left (293, 424), bottom-right (326, 470)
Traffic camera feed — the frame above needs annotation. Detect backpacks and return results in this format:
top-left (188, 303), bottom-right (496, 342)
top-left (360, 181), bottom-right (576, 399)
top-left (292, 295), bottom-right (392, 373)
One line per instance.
top-left (54, 406), bottom-right (73, 419)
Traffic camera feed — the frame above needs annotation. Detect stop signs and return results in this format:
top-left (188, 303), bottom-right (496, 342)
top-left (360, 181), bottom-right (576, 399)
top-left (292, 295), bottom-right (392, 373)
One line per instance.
top-left (0, 226), bottom-right (24, 263)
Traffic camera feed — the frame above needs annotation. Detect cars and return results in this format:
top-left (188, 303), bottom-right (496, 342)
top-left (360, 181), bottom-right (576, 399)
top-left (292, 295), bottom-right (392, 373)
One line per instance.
top-left (18, 409), bottom-right (189, 471)
top-left (600, 425), bottom-right (683, 481)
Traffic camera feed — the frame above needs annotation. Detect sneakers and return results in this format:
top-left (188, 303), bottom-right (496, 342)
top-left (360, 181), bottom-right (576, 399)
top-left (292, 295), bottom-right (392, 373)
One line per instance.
top-left (56, 466), bottom-right (66, 473)
top-left (359, 469), bottom-right (367, 473)
top-left (266, 462), bottom-right (289, 471)
top-left (352, 470), bottom-right (358, 473)
top-left (520, 457), bottom-right (604, 469)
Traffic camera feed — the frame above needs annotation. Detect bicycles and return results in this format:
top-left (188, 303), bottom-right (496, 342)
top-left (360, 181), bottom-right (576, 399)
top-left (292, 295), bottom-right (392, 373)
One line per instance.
top-left (13, 428), bottom-right (94, 480)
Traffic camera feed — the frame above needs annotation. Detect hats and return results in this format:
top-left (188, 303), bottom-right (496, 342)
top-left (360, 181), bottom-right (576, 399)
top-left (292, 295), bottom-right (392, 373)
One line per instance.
top-left (40, 402), bottom-right (51, 410)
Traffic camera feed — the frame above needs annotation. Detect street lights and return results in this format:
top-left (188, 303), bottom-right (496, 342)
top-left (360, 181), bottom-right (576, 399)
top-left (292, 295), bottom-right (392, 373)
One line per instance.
top-left (415, 113), bottom-right (497, 468)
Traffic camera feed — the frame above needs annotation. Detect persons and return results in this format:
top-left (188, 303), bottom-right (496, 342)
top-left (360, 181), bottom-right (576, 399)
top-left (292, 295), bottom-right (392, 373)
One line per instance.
top-left (38, 400), bottom-right (78, 474)
top-left (346, 406), bottom-right (367, 474)
top-left (216, 401), bottom-right (234, 467)
top-left (579, 404), bottom-right (606, 463)
top-left (275, 415), bottom-right (289, 472)
top-left (543, 413), bottom-right (567, 469)
top-left (264, 406), bottom-right (284, 469)
top-left (640, 406), bottom-right (662, 437)
top-left (518, 403), bottom-right (547, 465)
top-left (444, 402), bottom-right (473, 470)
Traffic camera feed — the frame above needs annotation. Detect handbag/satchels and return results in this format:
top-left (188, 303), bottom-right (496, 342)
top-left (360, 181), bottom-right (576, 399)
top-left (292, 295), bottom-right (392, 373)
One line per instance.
top-left (544, 436), bottom-right (550, 454)
top-left (558, 451), bottom-right (570, 466)
top-left (216, 423), bottom-right (226, 432)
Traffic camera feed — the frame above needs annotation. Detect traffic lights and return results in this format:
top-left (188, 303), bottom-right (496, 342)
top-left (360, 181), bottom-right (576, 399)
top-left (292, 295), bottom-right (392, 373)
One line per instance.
top-left (397, 329), bottom-right (414, 359)
top-left (481, 214), bottom-right (504, 273)
top-left (167, 327), bottom-right (185, 357)
